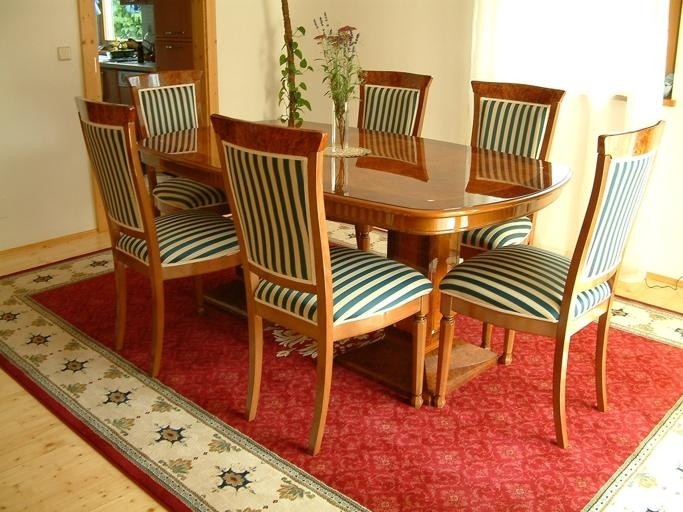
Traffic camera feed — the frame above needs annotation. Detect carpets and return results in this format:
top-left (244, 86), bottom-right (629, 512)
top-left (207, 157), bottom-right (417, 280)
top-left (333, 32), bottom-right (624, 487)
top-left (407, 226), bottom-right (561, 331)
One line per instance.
top-left (0, 220), bottom-right (683, 512)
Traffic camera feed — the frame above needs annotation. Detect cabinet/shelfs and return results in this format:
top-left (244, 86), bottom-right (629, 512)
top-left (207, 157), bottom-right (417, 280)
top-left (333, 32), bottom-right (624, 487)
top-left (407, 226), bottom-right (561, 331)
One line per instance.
top-left (154, 1), bottom-right (193, 70)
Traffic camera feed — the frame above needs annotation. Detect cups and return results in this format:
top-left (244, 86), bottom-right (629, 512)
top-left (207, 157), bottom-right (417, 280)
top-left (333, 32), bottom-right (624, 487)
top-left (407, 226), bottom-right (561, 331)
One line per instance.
top-left (331, 101), bottom-right (350, 153)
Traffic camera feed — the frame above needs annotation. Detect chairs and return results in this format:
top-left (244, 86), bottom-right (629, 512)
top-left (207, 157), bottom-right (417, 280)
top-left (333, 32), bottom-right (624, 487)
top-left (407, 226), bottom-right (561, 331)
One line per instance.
top-left (433, 120), bottom-right (665, 450)
top-left (456, 80), bottom-right (567, 365)
top-left (75, 94), bottom-right (241, 378)
top-left (132, 70), bottom-right (229, 217)
top-left (208, 113), bottom-right (433, 456)
top-left (358, 70), bottom-right (434, 137)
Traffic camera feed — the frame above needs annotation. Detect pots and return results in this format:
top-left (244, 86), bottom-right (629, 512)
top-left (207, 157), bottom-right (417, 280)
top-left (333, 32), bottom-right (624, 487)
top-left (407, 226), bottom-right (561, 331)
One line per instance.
top-left (111, 48), bottom-right (134, 58)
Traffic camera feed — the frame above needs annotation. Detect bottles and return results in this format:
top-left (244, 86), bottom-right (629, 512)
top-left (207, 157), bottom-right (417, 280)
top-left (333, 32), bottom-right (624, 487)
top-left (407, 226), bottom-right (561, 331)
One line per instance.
top-left (138, 42), bottom-right (144, 63)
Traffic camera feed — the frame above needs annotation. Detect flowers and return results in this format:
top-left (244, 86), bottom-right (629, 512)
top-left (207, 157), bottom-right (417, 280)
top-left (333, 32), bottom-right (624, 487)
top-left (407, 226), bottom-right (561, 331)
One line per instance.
top-left (312, 12), bottom-right (369, 146)
